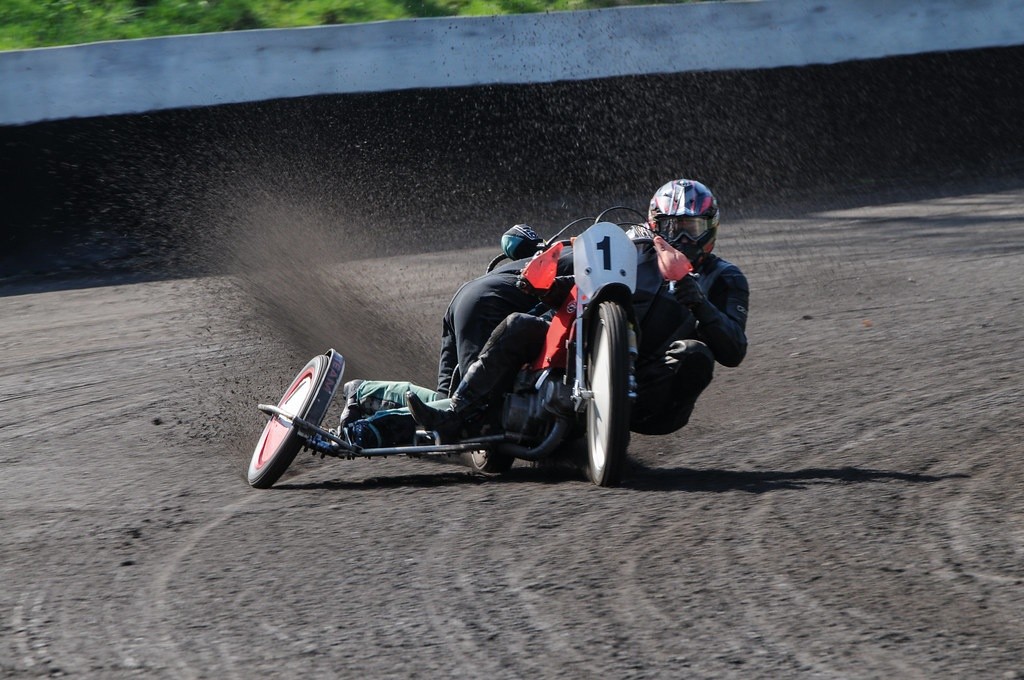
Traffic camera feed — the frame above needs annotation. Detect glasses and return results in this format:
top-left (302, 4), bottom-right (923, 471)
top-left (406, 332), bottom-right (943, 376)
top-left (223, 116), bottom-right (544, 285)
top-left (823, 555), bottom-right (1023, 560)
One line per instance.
top-left (653, 216), bottom-right (708, 243)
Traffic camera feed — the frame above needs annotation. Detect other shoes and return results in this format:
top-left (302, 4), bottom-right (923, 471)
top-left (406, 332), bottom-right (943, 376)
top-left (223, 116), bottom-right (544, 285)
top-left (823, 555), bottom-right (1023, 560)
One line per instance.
top-left (344, 418), bottom-right (378, 450)
top-left (338, 379), bottom-right (364, 439)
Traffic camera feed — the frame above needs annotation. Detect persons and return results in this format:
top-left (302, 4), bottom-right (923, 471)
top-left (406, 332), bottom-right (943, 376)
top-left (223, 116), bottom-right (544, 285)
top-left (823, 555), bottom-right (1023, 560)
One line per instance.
top-left (337, 219), bottom-right (655, 427)
top-left (405, 178), bottom-right (751, 437)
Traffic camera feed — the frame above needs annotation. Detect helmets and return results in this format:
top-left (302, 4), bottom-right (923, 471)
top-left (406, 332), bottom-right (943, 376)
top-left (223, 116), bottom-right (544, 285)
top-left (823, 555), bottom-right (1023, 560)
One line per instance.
top-left (647, 178), bottom-right (720, 266)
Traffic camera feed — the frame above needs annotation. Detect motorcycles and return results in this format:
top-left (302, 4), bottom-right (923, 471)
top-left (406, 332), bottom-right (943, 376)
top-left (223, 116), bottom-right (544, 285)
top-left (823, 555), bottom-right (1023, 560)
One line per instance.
top-left (246, 203), bottom-right (680, 490)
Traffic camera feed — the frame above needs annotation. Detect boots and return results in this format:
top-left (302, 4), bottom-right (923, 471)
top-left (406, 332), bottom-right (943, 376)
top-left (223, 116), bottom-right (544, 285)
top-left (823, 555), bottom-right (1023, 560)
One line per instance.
top-left (404, 357), bottom-right (502, 443)
